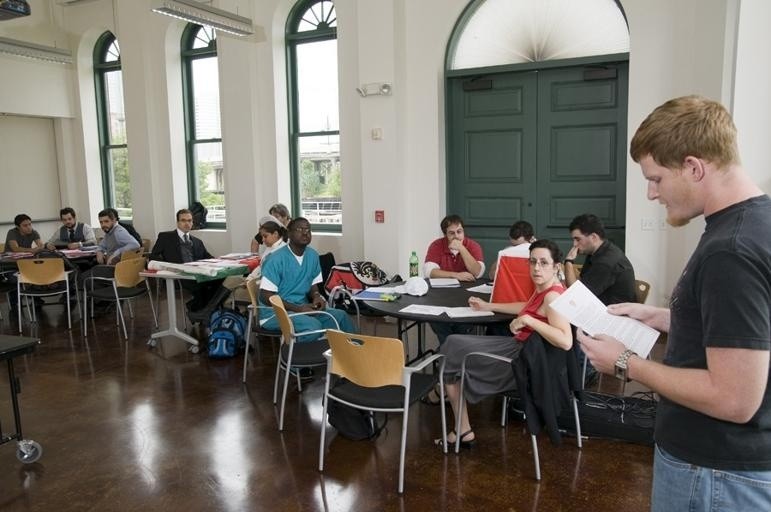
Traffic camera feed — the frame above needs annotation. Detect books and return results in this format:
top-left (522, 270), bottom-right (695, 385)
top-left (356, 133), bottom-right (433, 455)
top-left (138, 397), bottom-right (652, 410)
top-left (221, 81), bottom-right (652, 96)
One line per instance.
top-left (429, 278), bottom-right (460, 288)
top-left (466, 284), bottom-right (494, 294)
top-left (221, 252), bottom-right (256, 260)
top-left (350, 291), bottom-right (399, 302)
top-left (184, 258), bottom-right (235, 268)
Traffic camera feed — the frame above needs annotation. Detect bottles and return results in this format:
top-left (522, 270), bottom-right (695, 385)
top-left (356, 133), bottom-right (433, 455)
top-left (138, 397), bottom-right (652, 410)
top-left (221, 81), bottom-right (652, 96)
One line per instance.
top-left (409, 252), bottom-right (418, 277)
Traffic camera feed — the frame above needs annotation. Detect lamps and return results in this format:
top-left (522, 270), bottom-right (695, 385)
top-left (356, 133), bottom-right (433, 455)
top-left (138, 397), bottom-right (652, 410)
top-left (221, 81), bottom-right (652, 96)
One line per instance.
top-left (151, 1), bottom-right (254, 36)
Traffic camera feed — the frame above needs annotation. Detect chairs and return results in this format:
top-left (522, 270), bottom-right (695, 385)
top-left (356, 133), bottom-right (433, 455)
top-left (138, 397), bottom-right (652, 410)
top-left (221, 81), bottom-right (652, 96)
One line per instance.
top-left (14, 259), bottom-right (83, 331)
top-left (268, 295), bottom-right (342, 432)
top-left (89, 246), bottom-right (142, 328)
top-left (594, 280), bottom-right (650, 399)
top-left (154, 277), bottom-right (190, 331)
top-left (455, 352), bottom-right (584, 481)
top-left (243, 280), bottom-right (303, 391)
top-left (318, 252), bottom-right (409, 364)
top-left (83, 257), bottom-right (159, 339)
top-left (320, 330), bottom-right (449, 496)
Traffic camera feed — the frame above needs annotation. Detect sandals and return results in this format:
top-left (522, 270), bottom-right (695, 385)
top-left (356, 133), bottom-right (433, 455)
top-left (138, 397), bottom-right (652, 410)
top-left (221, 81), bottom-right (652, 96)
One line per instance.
top-left (420, 388), bottom-right (452, 407)
top-left (436, 427), bottom-right (475, 449)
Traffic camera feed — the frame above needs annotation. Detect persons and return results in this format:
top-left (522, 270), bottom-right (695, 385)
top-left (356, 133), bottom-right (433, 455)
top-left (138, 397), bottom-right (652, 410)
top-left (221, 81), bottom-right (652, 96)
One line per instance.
top-left (106, 208), bottom-right (143, 248)
top-left (79, 210), bottom-right (140, 291)
top-left (51, 208), bottom-right (97, 250)
top-left (149, 209), bottom-right (215, 325)
top-left (577, 94), bottom-right (770, 512)
top-left (564, 214), bottom-right (637, 307)
top-left (4, 214), bottom-right (55, 253)
top-left (251, 204), bottom-right (293, 252)
top-left (489, 221), bottom-right (537, 280)
top-left (424, 215), bottom-right (485, 345)
top-left (258, 218), bottom-right (357, 379)
top-left (420, 240), bottom-right (573, 449)
top-left (187, 221), bottom-right (289, 327)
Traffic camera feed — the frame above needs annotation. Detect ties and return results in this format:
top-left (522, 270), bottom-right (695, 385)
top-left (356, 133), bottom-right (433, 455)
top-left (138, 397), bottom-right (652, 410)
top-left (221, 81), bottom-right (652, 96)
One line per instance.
top-left (68, 229), bottom-right (75, 240)
top-left (183, 234), bottom-right (190, 246)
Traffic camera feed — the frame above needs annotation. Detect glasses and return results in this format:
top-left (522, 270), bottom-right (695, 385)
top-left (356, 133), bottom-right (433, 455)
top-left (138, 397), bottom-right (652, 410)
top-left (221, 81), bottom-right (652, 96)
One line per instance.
top-left (290, 226), bottom-right (313, 232)
top-left (529, 260), bottom-right (551, 266)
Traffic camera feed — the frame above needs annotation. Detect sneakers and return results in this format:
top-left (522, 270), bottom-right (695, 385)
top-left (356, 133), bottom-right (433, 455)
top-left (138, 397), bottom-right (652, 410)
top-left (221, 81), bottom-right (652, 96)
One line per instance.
top-left (279, 363), bottom-right (315, 381)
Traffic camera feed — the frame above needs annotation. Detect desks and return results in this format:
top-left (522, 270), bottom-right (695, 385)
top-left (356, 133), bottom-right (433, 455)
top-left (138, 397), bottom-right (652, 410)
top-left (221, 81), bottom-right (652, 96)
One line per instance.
top-left (0, 247), bottom-right (105, 266)
top-left (0, 336), bottom-right (43, 465)
top-left (363, 278), bottom-right (514, 373)
top-left (138, 254), bottom-right (259, 354)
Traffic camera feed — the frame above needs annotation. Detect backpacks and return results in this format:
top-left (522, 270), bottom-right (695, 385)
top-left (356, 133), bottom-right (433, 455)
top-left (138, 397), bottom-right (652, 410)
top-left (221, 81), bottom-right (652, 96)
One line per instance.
top-left (31, 250), bottom-right (75, 290)
top-left (322, 376), bottom-right (388, 441)
top-left (189, 202), bottom-right (208, 230)
top-left (209, 306), bottom-right (246, 360)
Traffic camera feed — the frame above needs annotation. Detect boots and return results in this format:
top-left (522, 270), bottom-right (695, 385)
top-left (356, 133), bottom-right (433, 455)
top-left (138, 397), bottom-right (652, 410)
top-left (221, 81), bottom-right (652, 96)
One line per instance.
top-left (186, 285), bottom-right (231, 325)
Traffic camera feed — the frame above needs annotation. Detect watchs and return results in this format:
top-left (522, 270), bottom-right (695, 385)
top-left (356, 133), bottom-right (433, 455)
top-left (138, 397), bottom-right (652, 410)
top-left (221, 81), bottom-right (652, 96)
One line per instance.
top-left (78, 240), bottom-right (84, 247)
top-left (312, 291), bottom-right (321, 295)
top-left (614, 350), bottom-right (638, 382)
